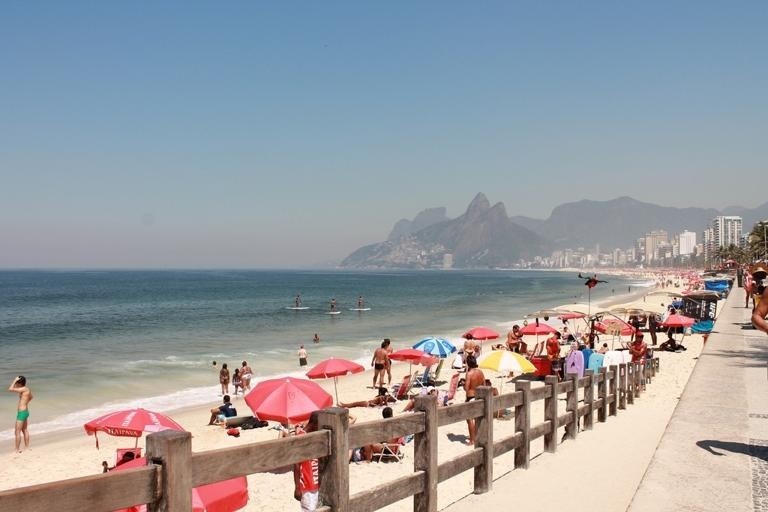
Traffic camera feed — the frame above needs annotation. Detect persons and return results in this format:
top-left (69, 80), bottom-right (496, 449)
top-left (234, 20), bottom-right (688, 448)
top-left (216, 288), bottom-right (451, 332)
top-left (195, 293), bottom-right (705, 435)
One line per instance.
top-left (643, 296), bottom-right (646, 302)
top-left (598, 343), bottom-right (610, 354)
top-left (463, 357), bottom-right (486, 446)
top-left (632, 270), bottom-right (704, 290)
top-left (563, 327), bottom-right (577, 341)
top-left (313, 334), bottom-right (321, 343)
top-left (612, 288), bottom-right (615, 296)
top-left (485, 379), bottom-right (506, 418)
top-left (737, 265), bottom-right (743, 287)
top-left (452, 348), bottom-right (463, 369)
top-left (463, 334), bottom-right (476, 372)
top-left (220, 363), bottom-right (230, 394)
top-left (297, 343), bottom-right (309, 365)
top-left (330, 298), bottom-right (336, 312)
top-left (628, 286), bottom-right (631, 293)
top-left (361, 407), bottom-right (397, 462)
top-left (627, 315), bottom-right (647, 329)
top-left (207, 395), bottom-right (238, 424)
top-left (212, 361), bottom-right (218, 369)
top-left (293, 410), bottom-right (320, 512)
top-left (743, 260), bottom-right (768, 334)
top-left (358, 296), bottom-right (362, 308)
top-left (658, 330), bottom-right (676, 350)
top-left (8, 375), bottom-right (33, 453)
top-left (579, 333), bottom-right (590, 345)
top-left (492, 342), bottom-right (508, 353)
top-left (648, 314), bottom-right (657, 346)
top-left (671, 296), bottom-right (677, 302)
top-left (238, 361), bottom-right (254, 391)
top-left (403, 389), bottom-right (439, 412)
top-left (295, 295), bottom-right (301, 307)
top-left (232, 368), bottom-right (244, 394)
top-left (371, 342), bottom-right (388, 388)
top-left (336, 387), bottom-right (388, 408)
top-left (546, 332), bottom-right (561, 360)
top-left (102, 451), bottom-right (141, 473)
top-left (506, 325), bottom-right (523, 353)
top-left (626, 332), bottom-right (647, 390)
top-left (383, 338), bottom-right (393, 385)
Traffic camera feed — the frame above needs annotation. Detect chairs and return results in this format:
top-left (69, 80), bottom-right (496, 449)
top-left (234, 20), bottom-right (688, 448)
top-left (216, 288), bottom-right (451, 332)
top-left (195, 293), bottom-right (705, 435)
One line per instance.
top-left (394, 375), bottom-right (411, 400)
top-left (412, 370), bottom-right (419, 393)
top-left (417, 361), bottom-right (432, 382)
top-left (371, 441), bottom-right (403, 465)
top-left (431, 357), bottom-right (444, 380)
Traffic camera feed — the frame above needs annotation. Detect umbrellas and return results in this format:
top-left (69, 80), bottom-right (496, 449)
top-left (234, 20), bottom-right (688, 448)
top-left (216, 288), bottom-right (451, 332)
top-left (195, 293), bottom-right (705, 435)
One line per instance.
top-left (83, 408), bottom-right (194, 459)
top-left (519, 322), bottom-right (558, 355)
top-left (462, 327), bottom-right (500, 354)
top-left (595, 319), bottom-right (636, 351)
top-left (304, 357), bottom-right (366, 403)
top-left (558, 312), bottom-right (585, 328)
top-left (661, 315), bottom-right (695, 339)
top-left (245, 377), bottom-right (334, 435)
top-left (477, 347), bottom-right (538, 395)
top-left (411, 336), bottom-right (457, 358)
top-left (388, 349), bottom-right (434, 389)
